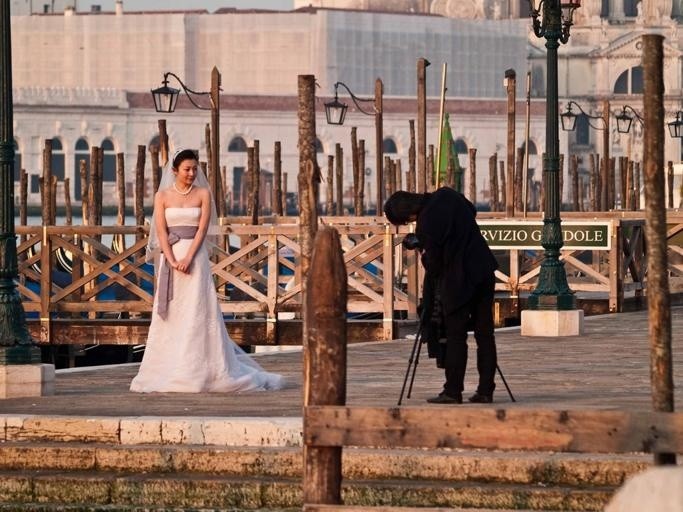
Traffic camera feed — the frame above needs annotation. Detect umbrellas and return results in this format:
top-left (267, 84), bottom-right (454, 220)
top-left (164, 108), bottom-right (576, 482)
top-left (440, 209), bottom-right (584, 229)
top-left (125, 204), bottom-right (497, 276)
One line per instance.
top-left (433, 114), bottom-right (463, 193)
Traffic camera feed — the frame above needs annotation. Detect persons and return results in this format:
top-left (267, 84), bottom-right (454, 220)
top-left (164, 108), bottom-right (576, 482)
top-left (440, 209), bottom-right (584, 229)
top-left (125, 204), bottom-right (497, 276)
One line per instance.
top-left (382, 186), bottom-right (499, 405)
top-left (129, 146), bottom-right (293, 394)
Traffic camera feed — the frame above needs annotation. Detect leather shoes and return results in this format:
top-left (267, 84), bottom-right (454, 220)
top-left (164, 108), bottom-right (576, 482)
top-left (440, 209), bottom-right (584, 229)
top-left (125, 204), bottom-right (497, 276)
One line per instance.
top-left (427, 389), bottom-right (462, 404)
top-left (469, 395), bottom-right (492, 403)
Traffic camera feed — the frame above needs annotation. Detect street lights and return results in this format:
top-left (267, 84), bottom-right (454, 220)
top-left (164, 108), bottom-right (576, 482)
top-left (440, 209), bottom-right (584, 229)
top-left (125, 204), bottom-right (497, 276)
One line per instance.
top-left (324, 77), bottom-right (384, 276)
top-left (668, 111), bottom-right (683, 162)
top-left (525, 0), bottom-right (581, 310)
top-left (560, 97), bottom-right (644, 211)
top-left (151, 65), bottom-right (225, 220)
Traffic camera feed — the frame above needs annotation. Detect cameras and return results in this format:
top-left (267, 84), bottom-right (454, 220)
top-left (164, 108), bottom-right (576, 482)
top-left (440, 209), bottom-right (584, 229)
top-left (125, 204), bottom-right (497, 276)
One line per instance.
top-left (403, 233), bottom-right (421, 251)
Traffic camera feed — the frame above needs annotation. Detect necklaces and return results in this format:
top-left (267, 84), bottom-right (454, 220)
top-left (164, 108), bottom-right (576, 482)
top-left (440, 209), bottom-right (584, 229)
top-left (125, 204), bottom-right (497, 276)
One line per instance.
top-left (173, 182), bottom-right (193, 195)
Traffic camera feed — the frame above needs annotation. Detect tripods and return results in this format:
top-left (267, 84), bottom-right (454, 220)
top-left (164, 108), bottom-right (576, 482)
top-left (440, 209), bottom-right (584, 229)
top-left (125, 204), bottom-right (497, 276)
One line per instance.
top-left (398, 310), bottom-right (516, 405)
